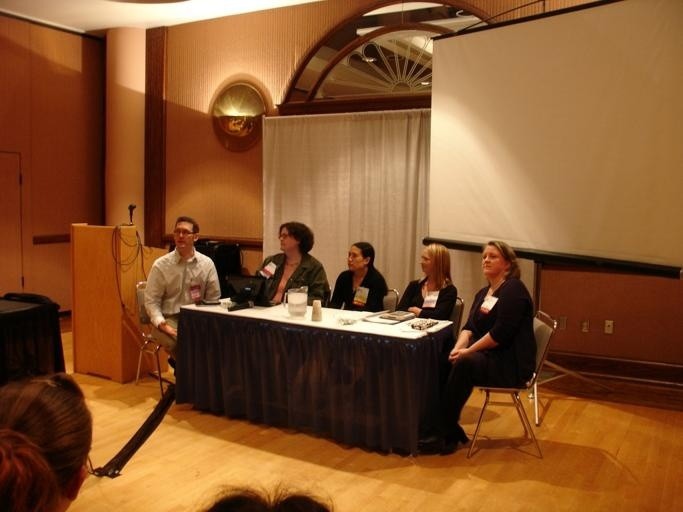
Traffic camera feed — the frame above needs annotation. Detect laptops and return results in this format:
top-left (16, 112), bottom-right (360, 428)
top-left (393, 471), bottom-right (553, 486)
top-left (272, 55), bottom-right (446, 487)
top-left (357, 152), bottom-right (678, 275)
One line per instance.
top-left (225, 273), bottom-right (282, 307)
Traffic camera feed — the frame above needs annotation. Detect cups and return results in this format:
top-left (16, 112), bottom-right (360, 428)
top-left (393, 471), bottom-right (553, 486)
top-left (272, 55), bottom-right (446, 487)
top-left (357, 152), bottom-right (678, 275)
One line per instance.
top-left (283, 285), bottom-right (308, 317)
top-left (311, 300), bottom-right (322, 322)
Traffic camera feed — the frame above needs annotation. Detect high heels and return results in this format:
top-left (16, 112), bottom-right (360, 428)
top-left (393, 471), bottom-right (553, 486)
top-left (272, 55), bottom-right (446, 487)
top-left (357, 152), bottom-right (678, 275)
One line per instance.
top-left (417, 420), bottom-right (470, 457)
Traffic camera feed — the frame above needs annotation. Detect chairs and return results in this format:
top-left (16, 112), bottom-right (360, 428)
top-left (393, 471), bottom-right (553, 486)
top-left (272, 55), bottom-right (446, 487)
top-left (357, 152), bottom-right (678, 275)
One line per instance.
top-left (323, 286), bottom-right (331, 307)
top-left (449, 297), bottom-right (464, 341)
top-left (382, 289), bottom-right (400, 312)
top-left (135, 281), bottom-right (170, 400)
top-left (467, 310), bottom-right (558, 461)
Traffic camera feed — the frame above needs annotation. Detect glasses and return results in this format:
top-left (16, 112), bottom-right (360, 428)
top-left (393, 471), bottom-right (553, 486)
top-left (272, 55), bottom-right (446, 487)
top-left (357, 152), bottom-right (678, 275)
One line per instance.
top-left (173, 229), bottom-right (194, 236)
top-left (278, 233), bottom-right (290, 240)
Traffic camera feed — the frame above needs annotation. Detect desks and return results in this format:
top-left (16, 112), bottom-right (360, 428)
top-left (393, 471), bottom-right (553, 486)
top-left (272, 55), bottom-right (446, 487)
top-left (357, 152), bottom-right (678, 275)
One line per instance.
top-left (175, 299), bottom-right (454, 457)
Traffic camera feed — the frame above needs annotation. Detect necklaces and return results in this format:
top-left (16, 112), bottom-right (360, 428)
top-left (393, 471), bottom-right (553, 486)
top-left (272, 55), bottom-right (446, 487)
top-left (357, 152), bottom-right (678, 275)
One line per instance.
top-left (284, 262), bottom-right (301, 268)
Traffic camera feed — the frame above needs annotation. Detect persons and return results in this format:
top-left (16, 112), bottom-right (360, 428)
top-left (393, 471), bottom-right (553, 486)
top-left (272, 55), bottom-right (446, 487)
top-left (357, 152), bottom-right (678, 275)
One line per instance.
top-left (395, 243), bottom-right (458, 321)
top-left (248, 221), bottom-right (332, 307)
top-left (0, 368), bottom-right (93, 511)
top-left (142, 215), bottom-right (222, 375)
top-left (415, 239), bottom-right (538, 457)
top-left (328, 241), bottom-right (388, 313)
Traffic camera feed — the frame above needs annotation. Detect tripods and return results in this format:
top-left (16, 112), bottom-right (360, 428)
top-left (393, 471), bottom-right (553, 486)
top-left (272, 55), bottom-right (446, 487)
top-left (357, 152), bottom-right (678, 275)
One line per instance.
top-left (526, 263), bottom-right (615, 427)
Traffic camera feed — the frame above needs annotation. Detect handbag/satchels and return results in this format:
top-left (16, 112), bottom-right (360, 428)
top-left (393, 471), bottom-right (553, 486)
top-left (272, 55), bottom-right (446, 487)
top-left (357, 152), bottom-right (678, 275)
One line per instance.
top-left (224, 275), bottom-right (268, 303)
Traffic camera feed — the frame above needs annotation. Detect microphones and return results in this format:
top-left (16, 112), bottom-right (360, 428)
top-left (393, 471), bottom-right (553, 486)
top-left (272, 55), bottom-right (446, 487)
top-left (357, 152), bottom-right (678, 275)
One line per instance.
top-left (128, 204), bottom-right (136, 225)
top-left (228, 301), bottom-right (254, 311)
